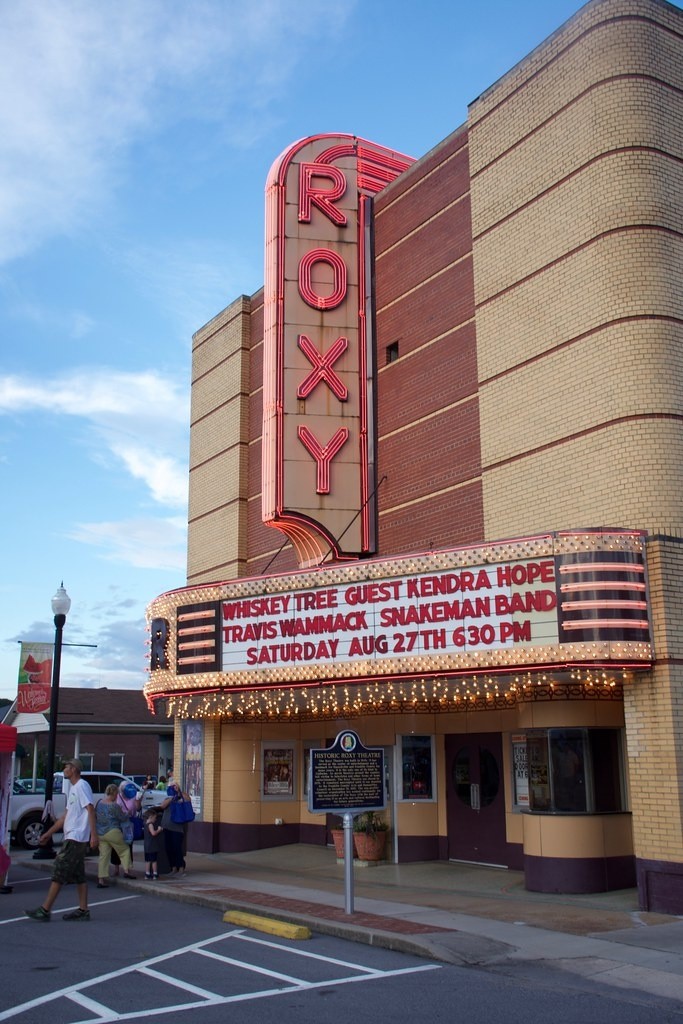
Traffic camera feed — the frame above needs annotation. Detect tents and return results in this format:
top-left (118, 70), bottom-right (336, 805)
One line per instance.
top-left (0, 723), bottom-right (17, 886)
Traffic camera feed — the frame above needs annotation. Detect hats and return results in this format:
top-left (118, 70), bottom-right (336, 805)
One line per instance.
top-left (64, 758), bottom-right (84, 771)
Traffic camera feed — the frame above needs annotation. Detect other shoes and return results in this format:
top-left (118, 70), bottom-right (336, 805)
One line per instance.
top-left (62, 909), bottom-right (91, 921)
top-left (22, 906), bottom-right (52, 922)
top-left (98, 884), bottom-right (110, 888)
top-left (123, 873), bottom-right (138, 879)
top-left (168, 866), bottom-right (186, 873)
top-left (152, 873), bottom-right (159, 880)
top-left (0, 885), bottom-right (14, 894)
top-left (144, 873), bottom-right (151, 881)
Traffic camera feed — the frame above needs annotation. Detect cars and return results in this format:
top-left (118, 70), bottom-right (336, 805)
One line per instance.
top-left (13, 779), bottom-right (47, 794)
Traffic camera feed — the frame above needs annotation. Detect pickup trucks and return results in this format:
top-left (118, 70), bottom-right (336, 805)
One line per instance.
top-left (9, 770), bottom-right (145, 851)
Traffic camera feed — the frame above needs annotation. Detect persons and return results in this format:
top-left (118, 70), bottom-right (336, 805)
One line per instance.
top-left (94, 781), bottom-right (143, 888)
top-left (0, 845), bottom-right (14, 894)
top-left (22, 758), bottom-right (98, 921)
top-left (143, 780), bottom-right (192, 880)
top-left (142, 767), bottom-right (174, 791)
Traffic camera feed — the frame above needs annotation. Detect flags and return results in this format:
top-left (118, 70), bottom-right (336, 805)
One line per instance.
top-left (16, 642), bottom-right (54, 713)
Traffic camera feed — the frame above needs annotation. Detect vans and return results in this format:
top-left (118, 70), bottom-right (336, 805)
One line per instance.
top-left (126, 775), bottom-right (159, 792)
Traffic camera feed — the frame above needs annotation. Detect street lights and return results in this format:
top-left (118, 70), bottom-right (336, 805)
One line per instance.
top-left (31, 580), bottom-right (71, 859)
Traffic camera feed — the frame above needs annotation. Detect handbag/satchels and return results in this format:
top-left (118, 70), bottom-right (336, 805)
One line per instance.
top-left (170, 793), bottom-right (195, 824)
top-left (120, 821), bottom-right (134, 844)
top-left (127, 817), bottom-right (145, 841)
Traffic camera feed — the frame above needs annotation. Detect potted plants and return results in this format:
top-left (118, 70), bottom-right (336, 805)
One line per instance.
top-left (330, 823), bottom-right (358, 858)
top-left (353, 811), bottom-right (389, 861)
